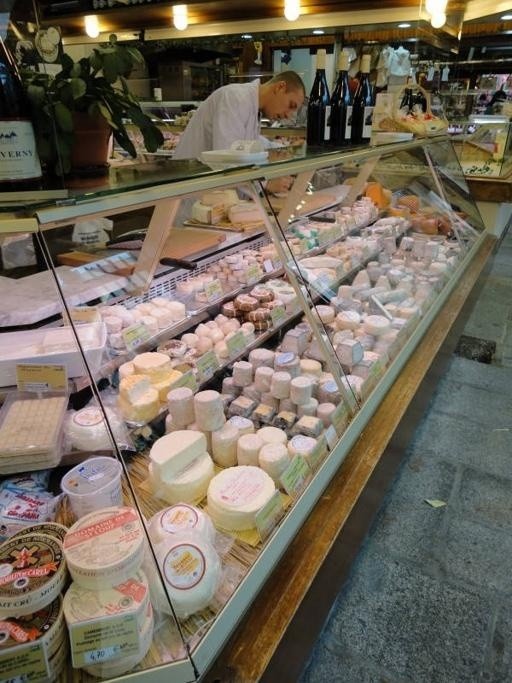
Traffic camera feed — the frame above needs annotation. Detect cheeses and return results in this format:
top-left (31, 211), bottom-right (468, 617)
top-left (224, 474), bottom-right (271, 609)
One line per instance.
top-left (0, 186), bottom-right (463, 683)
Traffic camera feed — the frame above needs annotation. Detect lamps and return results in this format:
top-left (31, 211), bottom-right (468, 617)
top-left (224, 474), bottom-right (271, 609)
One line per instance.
top-left (82, 12), bottom-right (101, 40)
top-left (171, 4), bottom-right (189, 31)
top-left (283, 0), bottom-right (300, 22)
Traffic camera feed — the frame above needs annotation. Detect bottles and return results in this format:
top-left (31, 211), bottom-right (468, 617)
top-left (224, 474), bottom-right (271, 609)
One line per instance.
top-left (351, 54), bottom-right (375, 145)
top-left (306, 48), bottom-right (333, 152)
top-left (399, 85), bottom-right (414, 119)
top-left (0, 61), bottom-right (58, 193)
top-left (330, 51), bottom-right (354, 148)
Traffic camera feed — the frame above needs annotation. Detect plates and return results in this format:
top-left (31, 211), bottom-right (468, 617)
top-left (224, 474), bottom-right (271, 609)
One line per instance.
top-left (198, 145), bottom-right (269, 167)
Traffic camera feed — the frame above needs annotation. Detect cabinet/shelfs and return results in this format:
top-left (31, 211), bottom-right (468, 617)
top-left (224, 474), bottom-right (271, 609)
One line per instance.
top-left (0, 116), bottom-right (510, 682)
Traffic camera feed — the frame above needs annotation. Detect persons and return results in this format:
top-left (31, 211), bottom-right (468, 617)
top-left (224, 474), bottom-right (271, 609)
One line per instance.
top-left (170, 69), bottom-right (305, 227)
top-left (484, 90), bottom-right (507, 115)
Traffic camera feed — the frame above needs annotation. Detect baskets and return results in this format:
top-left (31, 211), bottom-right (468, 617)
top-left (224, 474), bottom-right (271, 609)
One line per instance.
top-left (379, 112), bottom-right (448, 137)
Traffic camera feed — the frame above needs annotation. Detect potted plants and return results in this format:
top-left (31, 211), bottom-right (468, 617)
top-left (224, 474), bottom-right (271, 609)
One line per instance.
top-left (20, 34), bottom-right (165, 180)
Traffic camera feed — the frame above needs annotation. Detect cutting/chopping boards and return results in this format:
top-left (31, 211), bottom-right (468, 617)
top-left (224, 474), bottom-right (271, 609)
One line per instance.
top-left (54, 225), bottom-right (227, 278)
top-left (243, 193), bottom-right (338, 218)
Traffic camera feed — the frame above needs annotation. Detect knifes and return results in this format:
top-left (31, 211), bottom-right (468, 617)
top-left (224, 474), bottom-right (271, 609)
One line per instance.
top-left (72, 247), bottom-right (199, 271)
